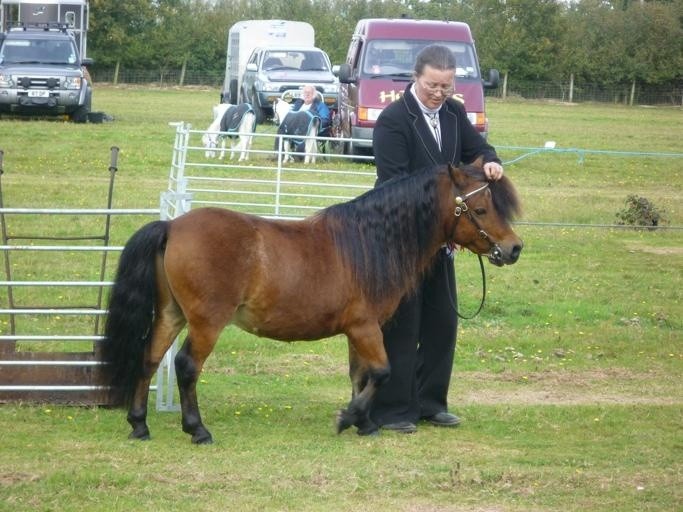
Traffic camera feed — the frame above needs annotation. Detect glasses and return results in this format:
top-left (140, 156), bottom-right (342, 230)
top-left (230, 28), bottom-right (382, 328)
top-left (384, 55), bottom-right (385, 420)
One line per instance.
top-left (422, 81), bottom-right (456, 96)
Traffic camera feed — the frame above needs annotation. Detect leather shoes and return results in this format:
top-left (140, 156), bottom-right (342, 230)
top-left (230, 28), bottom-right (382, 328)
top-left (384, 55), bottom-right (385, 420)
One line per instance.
top-left (381, 422), bottom-right (417, 432)
top-left (431, 412), bottom-right (462, 425)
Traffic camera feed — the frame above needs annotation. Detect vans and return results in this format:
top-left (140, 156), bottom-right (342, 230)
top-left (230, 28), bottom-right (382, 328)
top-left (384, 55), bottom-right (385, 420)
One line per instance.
top-left (337, 16), bottom-right (501, 163)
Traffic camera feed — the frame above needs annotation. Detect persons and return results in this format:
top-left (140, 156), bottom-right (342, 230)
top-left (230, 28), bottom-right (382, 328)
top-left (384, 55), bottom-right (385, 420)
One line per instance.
top-left (371, 42), bottom-right (505, 433)
top-left (276, 83), bottom-right (332, 161)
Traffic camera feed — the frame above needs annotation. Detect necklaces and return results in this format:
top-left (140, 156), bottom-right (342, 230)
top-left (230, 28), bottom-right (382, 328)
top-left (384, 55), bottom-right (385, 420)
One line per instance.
top-left (423, 109), bottom-right (445, 144)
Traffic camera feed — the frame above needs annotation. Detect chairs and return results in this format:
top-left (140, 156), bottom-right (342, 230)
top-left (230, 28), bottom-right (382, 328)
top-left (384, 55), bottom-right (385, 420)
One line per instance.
top-left (281, 90), bottom-right (332, 162)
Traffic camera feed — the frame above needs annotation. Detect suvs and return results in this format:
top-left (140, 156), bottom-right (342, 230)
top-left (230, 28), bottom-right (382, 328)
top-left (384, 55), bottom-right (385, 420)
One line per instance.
top-left (2, 27), bottom-right (91, 123)
top-left (237, 46), bottom-right (340, 118)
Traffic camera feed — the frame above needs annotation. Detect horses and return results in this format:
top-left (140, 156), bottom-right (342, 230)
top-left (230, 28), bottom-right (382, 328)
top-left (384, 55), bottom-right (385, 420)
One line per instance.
top-left (96, 153), bottom-right (525, 445)
top-left (272, 96), bottom-right (320, 164)
top-left (202, 103), bottom-right (256, 162)
